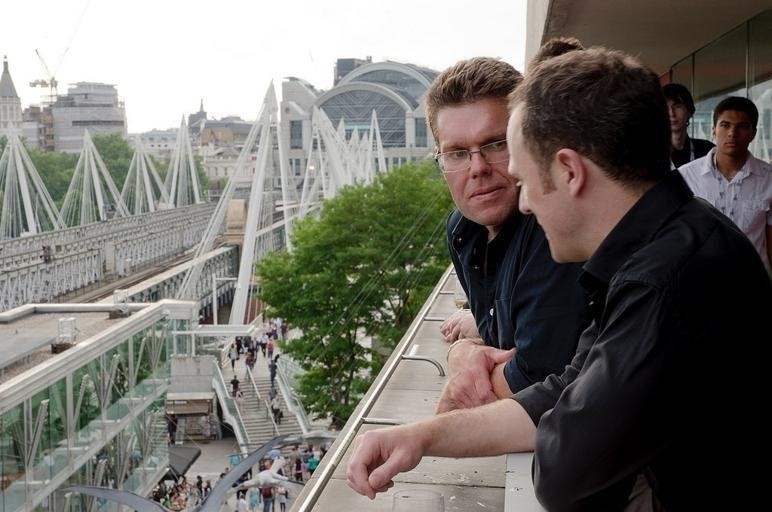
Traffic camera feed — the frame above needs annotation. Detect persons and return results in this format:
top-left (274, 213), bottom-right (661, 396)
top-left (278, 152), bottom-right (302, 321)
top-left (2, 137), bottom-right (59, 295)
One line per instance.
top-left (424, 57), bottom-right (595, 415)
top-left (343, 46), bottom-right (771, 510)
top-left (679, 97), bottom-right (771, 278)
top-left (149, 442), bottom-right (335, 512)
top-left (526, 36), bottom-right (587, 71)
top-left (438, 306), bottom-right (480, 342)
top-left (661, 83), bottom-right (716, 168)
top-left (226, 315), bottom-right (289, 424)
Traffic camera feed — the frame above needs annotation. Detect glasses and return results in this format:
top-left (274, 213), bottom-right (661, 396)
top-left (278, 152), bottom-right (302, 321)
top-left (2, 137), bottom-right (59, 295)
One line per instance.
top-left (435, 139), bottom-right (510, 176)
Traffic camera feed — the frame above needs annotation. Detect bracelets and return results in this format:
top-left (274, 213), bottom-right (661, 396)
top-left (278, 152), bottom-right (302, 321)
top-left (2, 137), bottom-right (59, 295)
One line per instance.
top-left (445, 340), bottom-right (463, 363)
